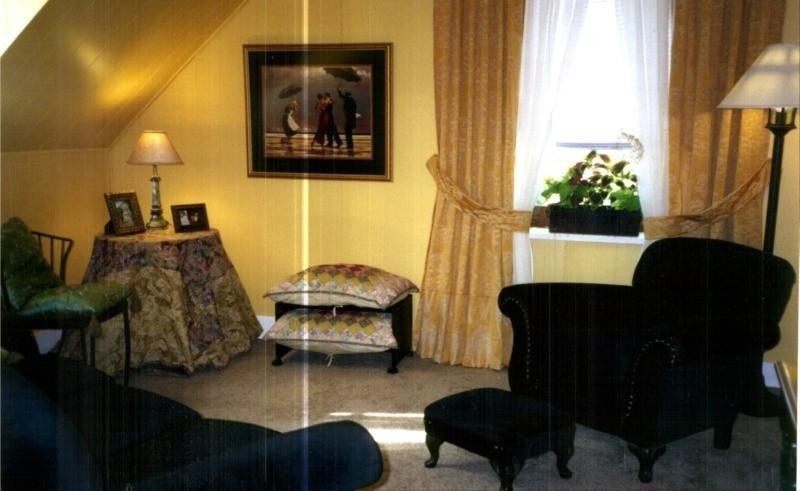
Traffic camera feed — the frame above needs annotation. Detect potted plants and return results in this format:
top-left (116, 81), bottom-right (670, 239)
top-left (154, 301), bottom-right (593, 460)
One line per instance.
top-left (539, 149), bottom-right (645, 238)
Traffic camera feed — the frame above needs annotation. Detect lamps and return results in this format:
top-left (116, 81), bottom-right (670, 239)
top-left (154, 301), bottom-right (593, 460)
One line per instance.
top-left (713, 40), bottom-right (799, 419)
top-left (124, 129), bottom-right (184, 233)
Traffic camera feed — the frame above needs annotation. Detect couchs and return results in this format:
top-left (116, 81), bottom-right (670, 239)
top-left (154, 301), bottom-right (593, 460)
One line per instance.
top-left (420, 383), bottom-right (575, 491)
top-left (494, 233), bottom-right (795, 484)
top-left (0, 352), bottom-right (386, 490)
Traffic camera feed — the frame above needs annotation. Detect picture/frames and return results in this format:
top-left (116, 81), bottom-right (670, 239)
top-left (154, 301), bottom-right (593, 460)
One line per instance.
top-left (238, 39), bottom-right (393, 185)
top-left (101, 189), bottom-right (146, 239)
top-left (169, 202), bottom-right (211, 234)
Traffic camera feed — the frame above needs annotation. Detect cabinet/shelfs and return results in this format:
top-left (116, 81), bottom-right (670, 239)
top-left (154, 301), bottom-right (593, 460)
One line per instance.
top-left (271, 291), bottom-right (419, 377)
top-left (525, 225), bottom-right (646, 294)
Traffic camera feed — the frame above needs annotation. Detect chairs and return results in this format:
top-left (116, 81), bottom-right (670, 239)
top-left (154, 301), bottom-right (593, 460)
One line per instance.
top-left (1, 217), bottom-right (133, 381)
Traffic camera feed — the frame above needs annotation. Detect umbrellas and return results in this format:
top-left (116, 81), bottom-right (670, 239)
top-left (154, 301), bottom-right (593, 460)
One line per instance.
top-left (278, 85), bottom-right (303, 101)
top-left (323, 66), bottom-right (362, 87)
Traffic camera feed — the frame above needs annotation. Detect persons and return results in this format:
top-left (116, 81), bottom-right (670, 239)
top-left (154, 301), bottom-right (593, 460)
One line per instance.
top-left (279, 99), bottom-right (301, 145)
top-left (337, 85), bottom-right (359, 150)
top-left (311, 94), bottom-right (330, 147)
top-left (323, 93), bottom-right (344, 149)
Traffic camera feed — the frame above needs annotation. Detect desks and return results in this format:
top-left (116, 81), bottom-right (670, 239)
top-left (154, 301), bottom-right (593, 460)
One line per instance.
top-left (55, 227), bottom-right (265, 378)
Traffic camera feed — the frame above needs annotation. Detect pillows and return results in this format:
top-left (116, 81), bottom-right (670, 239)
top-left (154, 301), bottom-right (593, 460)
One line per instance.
top-left (260, 261), bottom-right (422, 312)
top-left (256, 308), bottom-right (400, 357)
